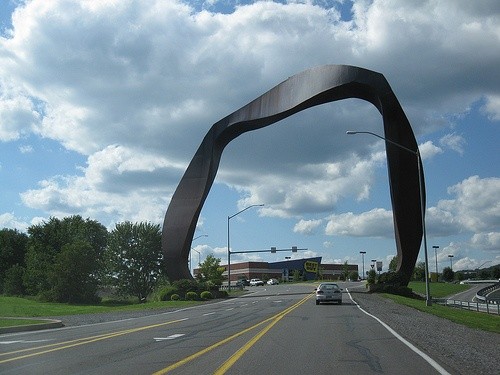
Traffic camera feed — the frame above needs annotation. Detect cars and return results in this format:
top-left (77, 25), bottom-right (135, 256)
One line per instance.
top-left (249, 279), bottom-right (264, 286)
top-left (315, 283), bottom-right (344, 305)
top-left (236, 280), bottom-right (250, 286)
top-left (267, 278), bottom-right (279, 285)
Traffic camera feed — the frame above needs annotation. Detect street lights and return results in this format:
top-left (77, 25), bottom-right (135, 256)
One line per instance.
top-left (360, 251), bottom-right (366, 280)
top-left (433, 245), bottom-right (439, 283)
top-left (227, 204), bottom-right (265, 294)
top-left (448, 255), bottom-right (454, 271)
top-left (371, 260), bottom-right (376, 268)
top-left (189, 234), bottom-right (209, 273)
top-left (346, 129), bottom-right (435, 307)
